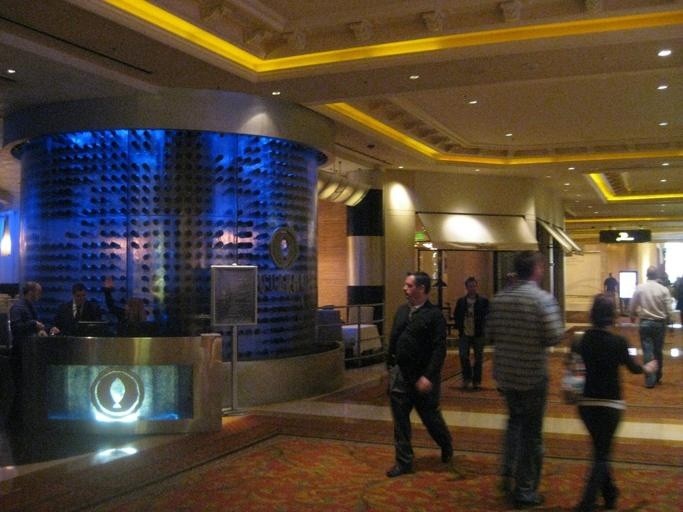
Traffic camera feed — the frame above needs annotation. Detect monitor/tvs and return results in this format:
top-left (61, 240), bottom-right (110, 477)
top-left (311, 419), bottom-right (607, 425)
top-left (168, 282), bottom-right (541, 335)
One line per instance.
top-left (73, 320), bottom-right (110, 337)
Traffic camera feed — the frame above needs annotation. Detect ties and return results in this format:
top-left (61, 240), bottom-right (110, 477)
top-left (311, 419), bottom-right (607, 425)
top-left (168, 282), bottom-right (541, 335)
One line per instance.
top-left (73, 305), bottom-right (82, 322)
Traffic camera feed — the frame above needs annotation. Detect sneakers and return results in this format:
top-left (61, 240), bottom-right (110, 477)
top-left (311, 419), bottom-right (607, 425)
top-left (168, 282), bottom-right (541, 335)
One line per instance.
top-left (384, 461), bottom-right (414, 478)
top-left (510, 490), bottom-right (548, 509)
top-left (439, 442), bottom-right (455, 465)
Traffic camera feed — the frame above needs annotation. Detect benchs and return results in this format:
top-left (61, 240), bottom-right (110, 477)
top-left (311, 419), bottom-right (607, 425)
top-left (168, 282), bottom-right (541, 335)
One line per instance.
top-left (316, 309), bottom-right (344, 343)
top-left (441, 307), bottom-right (456, 334)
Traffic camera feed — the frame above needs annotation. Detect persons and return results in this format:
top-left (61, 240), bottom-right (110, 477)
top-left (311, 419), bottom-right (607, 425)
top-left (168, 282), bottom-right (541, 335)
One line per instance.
top-left (627, 265), bottom-right (673, 388)
top-left (8, 281), bottom-right (61, 461)
top-left (567, 294), bottom-right (660, 512)
top-left (605, 273), bottom-right (617, 296)
top-left (54, 284), bottom-right (102, 335)
top-left (658, 271), bottom-right (671, 292)
top-left (672, 275), bottom-right (682, 326)
top-left (385, 271), bottom-right (453, 477)
top-left (103, 277), bottom-right (147, 337)
top-left (492, 251), bottom-right (567, 506)
top-left (454, 275), bottom-right (490, 392)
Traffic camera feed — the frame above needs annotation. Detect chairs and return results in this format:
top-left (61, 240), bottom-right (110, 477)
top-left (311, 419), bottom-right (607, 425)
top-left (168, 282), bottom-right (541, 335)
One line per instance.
top-left (349, 306), bottom-right (376, 323)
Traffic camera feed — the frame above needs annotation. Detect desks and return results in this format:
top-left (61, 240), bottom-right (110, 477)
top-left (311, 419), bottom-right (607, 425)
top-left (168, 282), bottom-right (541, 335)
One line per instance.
top-left (343, 325), bottom-right (383, 362)
